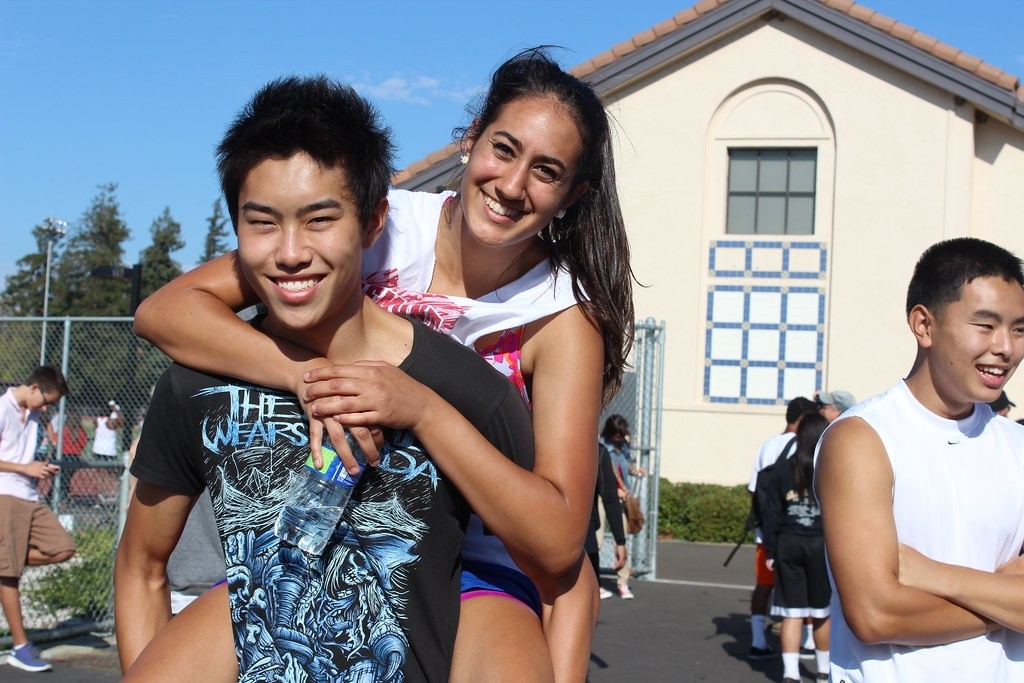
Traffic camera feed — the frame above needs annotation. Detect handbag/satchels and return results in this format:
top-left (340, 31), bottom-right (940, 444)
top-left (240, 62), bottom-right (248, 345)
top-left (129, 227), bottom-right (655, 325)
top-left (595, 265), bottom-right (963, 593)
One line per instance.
top-left (744, 436), bottom-right (797, 531)
top-left (625, 474), bottom-right (644, 533)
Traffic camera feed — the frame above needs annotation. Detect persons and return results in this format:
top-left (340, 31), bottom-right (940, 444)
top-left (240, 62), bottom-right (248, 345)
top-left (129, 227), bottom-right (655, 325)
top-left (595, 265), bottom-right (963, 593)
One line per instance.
top-left (814, 390), bottom-right (857, 424)
top-left (33, 399), bottom-right (148, 521)
top-left (0, 366), bottom-right (77, 674)
top-left (134, 42), bottom-right (652, 682)
top-left (812, 237), bottom-right (1024, 683)
top-left (750, 412), bottom-right (831, 683)
top-left (599, 415), bottom-right (646, 601)
top-left (111, 73), bottom-right (601, 683)
top-left (990, 390), bottom-right (1011, 418)
top-left (748, 396), bottom-right (817, 659)
top-left (585, 442), bottom-right (627, 588)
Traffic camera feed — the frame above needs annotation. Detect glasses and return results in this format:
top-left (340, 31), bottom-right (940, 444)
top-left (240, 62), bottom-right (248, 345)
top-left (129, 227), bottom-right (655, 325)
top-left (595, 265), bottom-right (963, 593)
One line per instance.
top-left (39, 386), bottom-right (52, 408)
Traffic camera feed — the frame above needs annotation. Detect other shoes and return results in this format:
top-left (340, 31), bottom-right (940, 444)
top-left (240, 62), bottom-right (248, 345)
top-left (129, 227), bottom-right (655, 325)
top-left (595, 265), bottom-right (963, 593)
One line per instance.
top-left (749, 645), bottom-right (776, 659)
top-left (799, 646), bottom-right (815, 659)
top-left (599, 587), bottom-right (613, 600)
top-left (782, 677), bottom-right (803, 683)
top-left (816, 673), bottom-right (829, 683)
top-left (618, 584), bottom-right (634, 599)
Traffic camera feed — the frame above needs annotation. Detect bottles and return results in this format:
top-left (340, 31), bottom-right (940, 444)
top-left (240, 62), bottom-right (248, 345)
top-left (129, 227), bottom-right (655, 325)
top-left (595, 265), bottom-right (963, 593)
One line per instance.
top-left (273, 387), bottom-right (386, 554)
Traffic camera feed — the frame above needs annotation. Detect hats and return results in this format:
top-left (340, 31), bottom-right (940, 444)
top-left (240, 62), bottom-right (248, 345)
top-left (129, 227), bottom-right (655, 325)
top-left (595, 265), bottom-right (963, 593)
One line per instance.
top-left (818, 391), bottom-right (857, 413)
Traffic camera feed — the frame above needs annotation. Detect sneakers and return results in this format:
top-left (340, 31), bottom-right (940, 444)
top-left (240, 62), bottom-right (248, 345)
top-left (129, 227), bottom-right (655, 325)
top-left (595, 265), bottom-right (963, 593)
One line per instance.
top-left (7, 641), bottom-right (52, 672)
